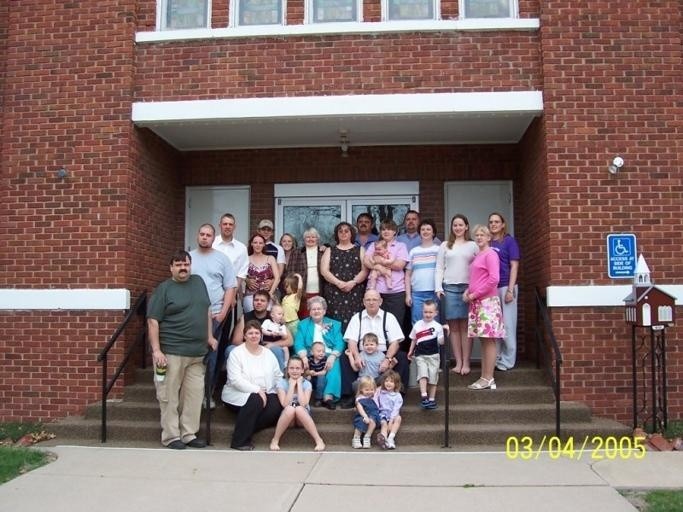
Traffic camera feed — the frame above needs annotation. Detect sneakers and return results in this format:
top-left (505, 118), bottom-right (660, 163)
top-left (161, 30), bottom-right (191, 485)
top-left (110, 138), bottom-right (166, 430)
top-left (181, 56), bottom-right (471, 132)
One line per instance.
top-left (203, 398), bottom-right (215, 409)
top-left (388, 438), bottom-right (396, 449)
top-left (429, 399), bottom-right (438, 410)
top-left (185, 439), bottom-right (207, 448)
top-left (166, 441), bottom-right (187, 449)
top-left (421, 399), bottom-right (430, 409)
top-left (376, 433), bottom-right (389, 451)
top-left (363, 437), bottom-right (371, 449)
top-left (322, 399), bottom-right (336, 409)
top-left (342, 397), bottom-right (354, 408)
top-left (352, 438), bottom-right (362, 449)
top-left (232, 445), bottom-right (254, 450)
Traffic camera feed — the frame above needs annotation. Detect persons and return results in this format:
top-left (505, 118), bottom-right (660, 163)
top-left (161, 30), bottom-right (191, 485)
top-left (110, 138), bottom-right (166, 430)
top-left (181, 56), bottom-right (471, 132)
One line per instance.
top-left (353, 213), bottom-right (378, 249)
top-left (260, 304), bottom-right (289, 367)
top-left (340, 289), bottom-right (410, 409)
top-left (320, 221), bottom-right (369, 335)
top-left (244, 219), bottom-right (287, 299)
top-left (220, 320), bottom-right (283, 451)
top-left (406, 299), bottom-right (450, 409)
top-left (286, 227), bottom-right (327, 319)
top-left (351, 375), bottom-right (380, 449)
top-left (462, 224), bottom-right (500, 390)
top-left (396, 211), bottom-right (441, 250)
top-left (187, 224), bottom-right (238, 410)
top-left (369, 240), bottom-right (395, 289)
top-left (363, 218), bottom-right (409, 330)
top-left (146, 249), bottom-right (219, 449)
top-left (404, 219), bottom-right (440, 326)
top-left (434, 214), bottom-right (480, 375)
top-left (211, 213), bottom-right (249, 371)
top-left (269, 271), bottom-right (303, 335)
top-left (302, 342), bottom-right (328, 407)
top-left (487, 212), bottom-right (520, 371)
top-left (373, 370), bottom-right (403, 450)
top-left (242, 233), bottom-right (281, 313)
top-left (345, 333), bottom-right (398, 386)
top-left (280, 233), bottom-right (296, 271)
top-left (291, 296), bottom-right (345, 410)
top-left (269, 356), bottom-right (326, 451)
top-left (222, 290), bottom-right (294, 378)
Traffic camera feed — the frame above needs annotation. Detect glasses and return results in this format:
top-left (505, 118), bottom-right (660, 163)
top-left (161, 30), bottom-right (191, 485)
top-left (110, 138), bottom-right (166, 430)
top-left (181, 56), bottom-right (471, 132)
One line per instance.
top-left (258, 228), bottom-right (273, 233)
top-left (338, 229), bottom-right (350, 233)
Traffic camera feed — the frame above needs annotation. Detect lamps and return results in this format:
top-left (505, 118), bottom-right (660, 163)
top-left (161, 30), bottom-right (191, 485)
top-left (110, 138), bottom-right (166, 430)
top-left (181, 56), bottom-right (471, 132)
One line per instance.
top-left (336, 129), bottom-right (352, 158)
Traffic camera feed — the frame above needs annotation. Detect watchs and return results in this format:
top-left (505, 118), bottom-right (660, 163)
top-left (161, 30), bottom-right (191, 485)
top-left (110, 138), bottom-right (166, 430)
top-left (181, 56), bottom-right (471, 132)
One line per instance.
top-left (507, 288), bottom-right (513, 293)
top-left (352, 279), bottom-right (357, 284)
top-left (385, 356), bottom-right (391, 361)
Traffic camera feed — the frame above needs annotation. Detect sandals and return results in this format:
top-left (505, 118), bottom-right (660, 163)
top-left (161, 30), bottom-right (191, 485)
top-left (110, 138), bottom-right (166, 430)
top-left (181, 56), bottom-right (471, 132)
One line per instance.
top-left (466, 378), bottom-right (497, 391)
